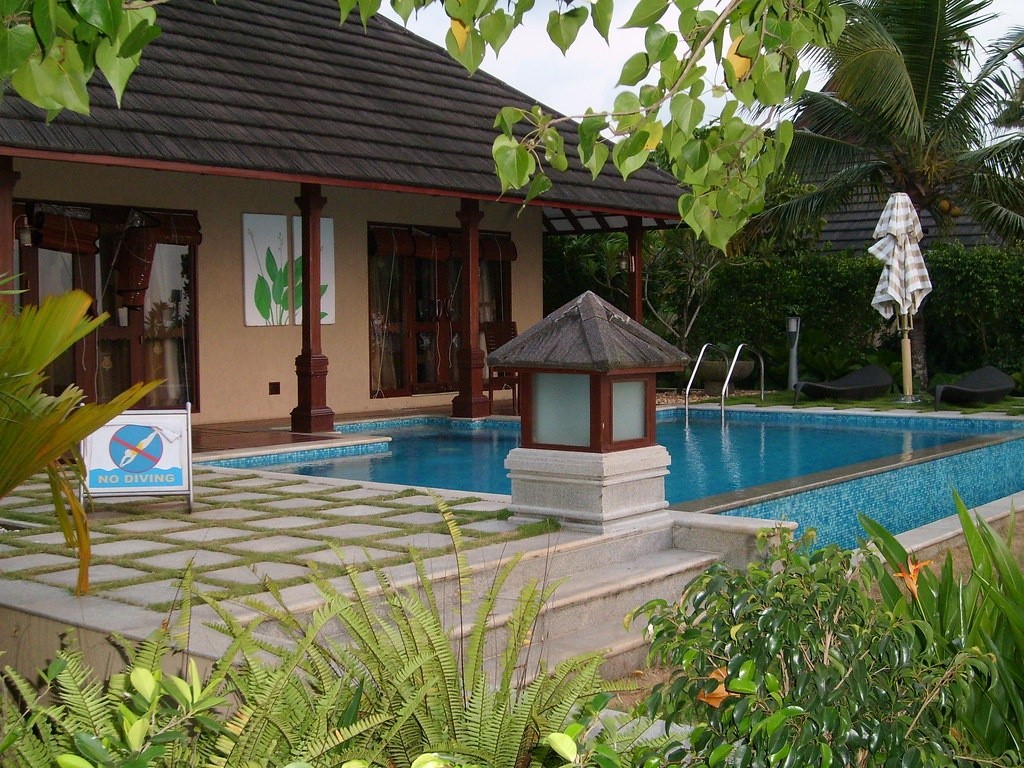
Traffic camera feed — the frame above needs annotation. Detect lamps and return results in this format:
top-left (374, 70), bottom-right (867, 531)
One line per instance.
top-left (13, 213), bottom-right (33, 252)
top-left (789, 318), bottom-right (797, 332)
top-left (619, 250), bottom-right (634, 272)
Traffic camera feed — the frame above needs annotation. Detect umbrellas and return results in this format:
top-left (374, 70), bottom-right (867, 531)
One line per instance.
top-left (867, 192), bottom-right (932, 395)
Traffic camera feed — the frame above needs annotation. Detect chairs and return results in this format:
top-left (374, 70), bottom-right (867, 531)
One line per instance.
top-left (483, 321), bottom-right (519, 415)
